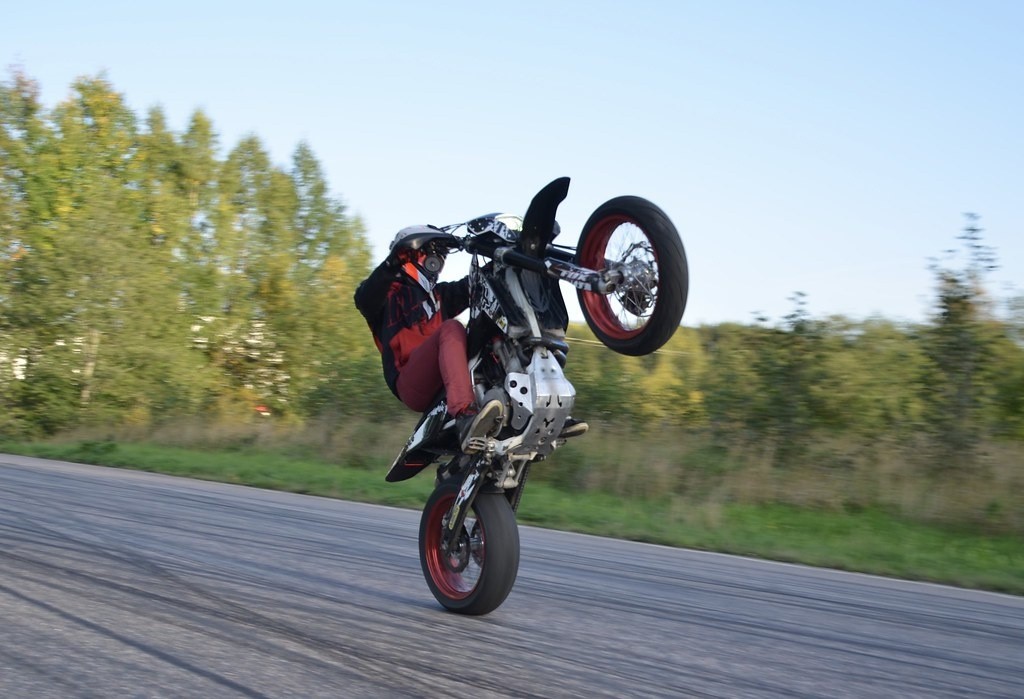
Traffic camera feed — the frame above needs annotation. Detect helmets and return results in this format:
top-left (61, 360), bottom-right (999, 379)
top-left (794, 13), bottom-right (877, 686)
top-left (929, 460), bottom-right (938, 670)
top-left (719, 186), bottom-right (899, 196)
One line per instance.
top-left (389, 225), bottom-right (450, 292)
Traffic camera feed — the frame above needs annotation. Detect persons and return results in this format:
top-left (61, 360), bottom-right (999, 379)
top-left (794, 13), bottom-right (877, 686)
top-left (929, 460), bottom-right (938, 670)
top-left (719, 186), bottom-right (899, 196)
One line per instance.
top-left (354, 224), bottom-right (588, 452)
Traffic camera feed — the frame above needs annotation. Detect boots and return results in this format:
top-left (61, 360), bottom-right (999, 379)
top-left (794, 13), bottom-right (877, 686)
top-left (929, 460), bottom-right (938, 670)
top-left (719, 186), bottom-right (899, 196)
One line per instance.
top-left (536, 416), bottom-right (589, 438)
top-left (454, 398), bottom-right (503, 455)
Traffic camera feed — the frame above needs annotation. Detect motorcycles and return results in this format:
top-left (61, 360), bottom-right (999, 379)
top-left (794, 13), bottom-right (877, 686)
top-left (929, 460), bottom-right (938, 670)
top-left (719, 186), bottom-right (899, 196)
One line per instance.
top-left (385, 177), bottom-right (689, 615)
